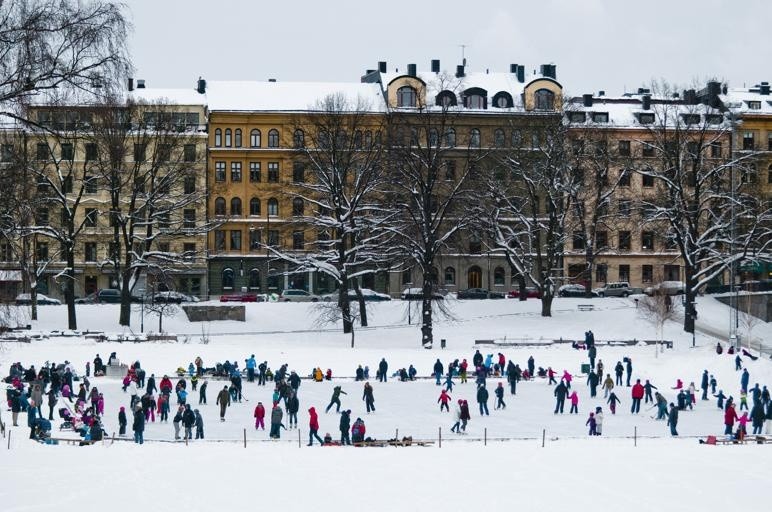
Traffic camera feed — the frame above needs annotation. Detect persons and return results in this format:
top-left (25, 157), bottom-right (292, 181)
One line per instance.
top-left (5, 330), bottom-right (771, 445)
top-left (664, 294), bottom-right (671, 312)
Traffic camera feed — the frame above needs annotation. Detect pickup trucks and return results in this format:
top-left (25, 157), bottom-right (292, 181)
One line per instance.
top-left (597, 281), bottom-right (634, 298)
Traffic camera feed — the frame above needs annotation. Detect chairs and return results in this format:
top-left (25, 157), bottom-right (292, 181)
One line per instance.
top-left (700, 435), bottom-right (717, 445)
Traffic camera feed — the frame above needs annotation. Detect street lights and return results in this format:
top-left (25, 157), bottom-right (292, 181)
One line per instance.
top-left (405, 281), bottom-right (413, 325)
top-left (735, 284), bottom-right (742, 354)
top-left (137, 289), bottom-right (147, 333)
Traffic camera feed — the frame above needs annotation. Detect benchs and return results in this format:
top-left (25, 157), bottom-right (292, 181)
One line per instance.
top-left (207, 370), bottom-right (247, 378)
top-left (716, 438), bottom-right (756, 444)
top-left (756, 435), bottom-right (772, 444)
top-left (49, 437), bottom-right (84, 447)
top-left (175, 370), bottom-right (198, 377)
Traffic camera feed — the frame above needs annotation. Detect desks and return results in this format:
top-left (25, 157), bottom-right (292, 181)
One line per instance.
top-left (105, 365), bottom-right (129, 377)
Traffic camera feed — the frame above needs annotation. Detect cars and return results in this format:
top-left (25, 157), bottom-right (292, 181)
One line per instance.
top-left (400, 288), bottom-right (446, 299)
top-left (644, 280), bottom-right (689, 296)
top-left (73, 287), bottom-right (203, 303)
top-left (557, 284), bottom-right (599, 297)
top-left (15, 293), bottom-right (63, 305)
top-left (457, 287), bottom-right (505, 299)
top-left (743, 279), bottom-right (762, 285)
top-left (279, 287), bottom-right (392, 302)
top-left (508, 286), bottom-right (545, 298)
